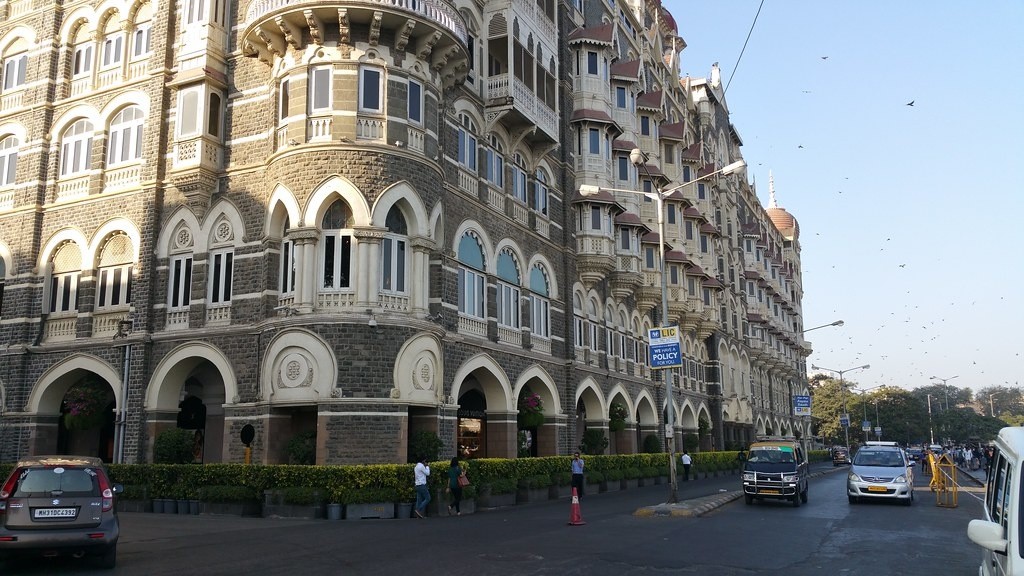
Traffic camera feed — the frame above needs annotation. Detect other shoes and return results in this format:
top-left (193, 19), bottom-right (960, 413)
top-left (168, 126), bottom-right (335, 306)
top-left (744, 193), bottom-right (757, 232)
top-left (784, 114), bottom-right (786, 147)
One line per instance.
top-left (457, 511), bottom-right (461, 516)
top-left (414, 509), bottom-right (423, 519)
top-left (448, 506), bottom-right (452, 515)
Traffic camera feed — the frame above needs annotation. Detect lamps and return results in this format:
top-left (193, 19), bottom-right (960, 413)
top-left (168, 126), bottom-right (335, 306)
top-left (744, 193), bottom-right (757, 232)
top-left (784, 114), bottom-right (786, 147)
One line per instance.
top-left (288, 138), bottom-right (298, 146)
top-left (395, 140), bottom-right (403, 147)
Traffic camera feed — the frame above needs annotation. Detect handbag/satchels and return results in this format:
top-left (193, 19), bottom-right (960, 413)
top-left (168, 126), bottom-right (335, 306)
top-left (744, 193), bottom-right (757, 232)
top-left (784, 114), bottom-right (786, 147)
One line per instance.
top-left (457, 475), bottom-right (469, 487)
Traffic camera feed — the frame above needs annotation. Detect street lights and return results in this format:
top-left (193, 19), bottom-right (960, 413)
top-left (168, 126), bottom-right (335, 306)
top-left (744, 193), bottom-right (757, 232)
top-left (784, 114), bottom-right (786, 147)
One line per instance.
top-left (858, 397), bottom-right (894, 441)
top-left (841, 383), bottom-right (886, 442)
top-left (811, 364), bottom-right (871, 465)
top-left (929, 375), bottom-right (960, 448)
top-left (579, 148), bottom-right (749, 496)
top-left (753, 320), bottom-right (845, 464)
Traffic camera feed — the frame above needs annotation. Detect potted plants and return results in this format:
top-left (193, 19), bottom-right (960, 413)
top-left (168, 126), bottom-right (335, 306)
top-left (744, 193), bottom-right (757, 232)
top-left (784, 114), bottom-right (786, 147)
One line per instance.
top-left (429, 460), bottom-right (740, 517)
top-left (325, 486), bottom-right (416, 522)
top-left (262, 484), bottom-right (323, 519)
top-left (114, 481), bottom-right (255, 517)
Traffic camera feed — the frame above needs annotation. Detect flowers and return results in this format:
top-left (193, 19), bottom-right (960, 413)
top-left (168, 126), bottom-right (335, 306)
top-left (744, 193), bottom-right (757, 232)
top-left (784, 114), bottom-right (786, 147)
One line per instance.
top-left (610, 401), bottom-right (628, 421)
top-left (64, 386), bottom-right (107, 416)
top-left (520, 390), bottom-right (546, 414)
top-left (698, 416), bottom-right (710, 429)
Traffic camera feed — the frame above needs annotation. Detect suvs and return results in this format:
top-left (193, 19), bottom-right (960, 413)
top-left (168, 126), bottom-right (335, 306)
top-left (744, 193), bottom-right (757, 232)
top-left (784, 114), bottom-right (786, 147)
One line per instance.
top-left (0, 455), bottom-right (124, 575)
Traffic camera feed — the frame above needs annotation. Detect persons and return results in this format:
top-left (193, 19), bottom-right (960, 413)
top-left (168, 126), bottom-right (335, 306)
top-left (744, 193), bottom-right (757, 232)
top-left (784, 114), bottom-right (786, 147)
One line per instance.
top-left (681, 450), bottom-right (692, 482)
top-left (921, 443), bottom-right (993, 474)
top-left (446, 457), bottom-right (467, 518)
top-left (414, 454), bottom-right (431, 519)
top-left (828, 448), bottom-right (837, 456)
top-left (738, 447), bottom-right (746, 476)
top-left (570, 452), bottom-right (584, 503)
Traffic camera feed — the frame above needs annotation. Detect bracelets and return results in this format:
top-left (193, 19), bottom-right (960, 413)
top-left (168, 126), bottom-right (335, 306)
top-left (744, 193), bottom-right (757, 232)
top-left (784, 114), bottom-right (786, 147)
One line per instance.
top-left (447, 487), bottom-right (450, 488)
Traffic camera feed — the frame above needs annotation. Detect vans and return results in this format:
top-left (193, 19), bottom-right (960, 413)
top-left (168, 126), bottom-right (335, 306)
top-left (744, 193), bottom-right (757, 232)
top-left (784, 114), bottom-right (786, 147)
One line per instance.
top-left (965, 426), bottom-right (1024, 576)
top-left (742, 434), bottom-right (809, 507)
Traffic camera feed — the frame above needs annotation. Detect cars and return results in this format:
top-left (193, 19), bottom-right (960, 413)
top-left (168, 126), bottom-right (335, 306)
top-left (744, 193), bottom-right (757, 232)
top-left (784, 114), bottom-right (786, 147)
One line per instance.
top-left (832, 447), bottom-right (851, 466)
top-left (844, 440), bottom-right (916, 507)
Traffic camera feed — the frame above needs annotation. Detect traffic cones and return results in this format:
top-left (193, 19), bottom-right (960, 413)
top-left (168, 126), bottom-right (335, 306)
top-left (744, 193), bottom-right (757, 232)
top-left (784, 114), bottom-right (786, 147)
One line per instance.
top-left (565, 486), bottom-right (587, 526)
